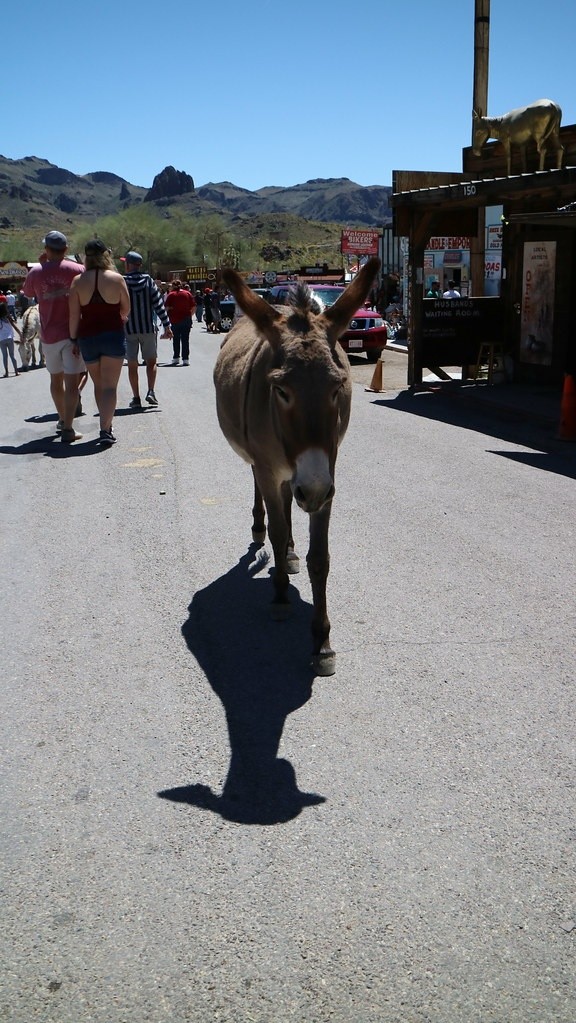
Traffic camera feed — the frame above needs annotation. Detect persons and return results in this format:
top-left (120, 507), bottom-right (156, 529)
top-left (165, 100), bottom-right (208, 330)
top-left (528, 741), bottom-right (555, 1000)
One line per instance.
top-left (121, 251), bottom-right (174, 410)
top-left (287, 274), bottom-right (298, 281)
top-left (0, 303), bottom-right (23, 377)
top-left (368, 272), bottom-right (400, 319)
top-left (22, 230), bottom-right (88, 443)
top-left (68, 239), bottom-right (131, 443)
top-left (427, 280), bottom-right (440, 297)
top-left (0, 290), bottom-right (28, 322)
top-left (155, 279), bottom-right (233, 334)
top-left (165, 280), bottom-right (196, 366)
top-left (443, 280), bottom-right (461, 299)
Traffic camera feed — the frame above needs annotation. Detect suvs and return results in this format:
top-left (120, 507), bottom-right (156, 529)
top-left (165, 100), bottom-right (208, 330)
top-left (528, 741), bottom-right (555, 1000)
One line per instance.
top-left (267, 284), bottom-right (387, 361)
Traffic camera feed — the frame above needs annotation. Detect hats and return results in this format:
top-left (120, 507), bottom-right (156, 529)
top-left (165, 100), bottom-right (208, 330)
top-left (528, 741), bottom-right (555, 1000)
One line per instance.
top-left (42, 231), bottom-right (67, 248)
top-left (120, 251), bottom-right (143, 266)
top-left (205, 288), bottom-right (213, 293)
top-left (7, 290), bottom-right (11, 293)
top-left (85, 240), bottom-right (107, 256)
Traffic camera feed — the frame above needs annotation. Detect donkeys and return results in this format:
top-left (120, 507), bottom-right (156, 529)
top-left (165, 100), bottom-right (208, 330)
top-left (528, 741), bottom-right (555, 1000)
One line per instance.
top-left (14, 303), bottom-right (46, 372)
top-left (212, 250), bottom-right (381, 677)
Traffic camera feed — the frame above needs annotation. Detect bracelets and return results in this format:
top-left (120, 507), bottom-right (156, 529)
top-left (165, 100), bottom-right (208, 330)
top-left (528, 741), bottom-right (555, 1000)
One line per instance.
top-left (70, 337), bottom-right (77, 344)
top-left (120, 312), bottom-right (129, 318)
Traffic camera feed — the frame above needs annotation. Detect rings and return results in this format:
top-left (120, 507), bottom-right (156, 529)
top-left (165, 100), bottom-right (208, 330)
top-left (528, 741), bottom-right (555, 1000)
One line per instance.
top-left (73, 353), bottom-right (77, 354)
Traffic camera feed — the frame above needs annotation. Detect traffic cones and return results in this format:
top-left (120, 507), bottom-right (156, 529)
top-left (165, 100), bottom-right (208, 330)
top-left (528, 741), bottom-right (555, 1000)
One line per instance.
top-left (545, 375), bottom-right (576, 447)
top-left (364, 359), bottom-right (386, 394)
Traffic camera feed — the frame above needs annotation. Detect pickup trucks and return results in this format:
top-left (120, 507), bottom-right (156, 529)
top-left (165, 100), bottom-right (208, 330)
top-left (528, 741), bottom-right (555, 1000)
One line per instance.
top-left (219, 289), bottom-right (270, 333)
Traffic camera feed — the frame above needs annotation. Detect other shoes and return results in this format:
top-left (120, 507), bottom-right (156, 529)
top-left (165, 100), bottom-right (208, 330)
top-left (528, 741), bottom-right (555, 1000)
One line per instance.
top-left (3, 373), bottom-right (8, 377)
top-left (16, 371), bottom-right (21, 376)
top-left (172, 358), bottom-right (180, 364)
top-left (183, 360), bottom-right (190, 365)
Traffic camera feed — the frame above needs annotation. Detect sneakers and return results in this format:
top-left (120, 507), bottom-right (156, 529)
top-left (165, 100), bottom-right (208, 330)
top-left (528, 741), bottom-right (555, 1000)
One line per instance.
top-left (100, 427), bottom-right (117, 443)
top-left (128, 397), bottom-right (141, 407)
top-left (145, 391), bottom-right (158, 404)
top-left (62, 429), bottom-right (83, 442)
top-left (56, 419), bottom-right (64, 428)
